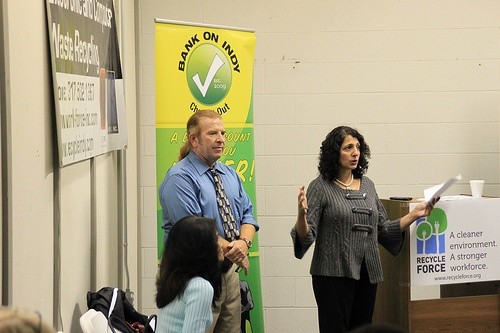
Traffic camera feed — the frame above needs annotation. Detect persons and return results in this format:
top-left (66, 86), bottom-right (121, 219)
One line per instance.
top-left (155, 215), bottom-right (225, 333)
top-left (158, 110), bottom-right (259, 333)
top-left (290, 126), bottom-right (441, 333)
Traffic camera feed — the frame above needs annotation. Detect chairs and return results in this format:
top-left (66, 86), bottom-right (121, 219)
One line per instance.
top-left (78, 289), bottom-right (146, 333)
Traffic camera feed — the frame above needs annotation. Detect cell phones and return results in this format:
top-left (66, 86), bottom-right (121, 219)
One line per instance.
top-left (390, 196), bottom-right (413, 201)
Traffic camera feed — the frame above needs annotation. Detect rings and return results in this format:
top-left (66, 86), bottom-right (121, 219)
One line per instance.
top-left (235, 257), bottom-right (239, 260)
top-left (298, 202), bottom-right (302, 206)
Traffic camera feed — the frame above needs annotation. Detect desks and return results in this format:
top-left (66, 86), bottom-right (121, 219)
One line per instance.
top-left (371, 195), bottom-right (500, 333)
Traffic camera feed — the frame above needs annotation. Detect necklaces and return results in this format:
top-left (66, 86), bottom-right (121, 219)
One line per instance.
top-left (336, 175), bottom-right (353, 187)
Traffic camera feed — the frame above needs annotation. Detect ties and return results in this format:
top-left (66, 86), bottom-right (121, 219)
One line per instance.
top-left (210, 169), bottom-right (241, 242)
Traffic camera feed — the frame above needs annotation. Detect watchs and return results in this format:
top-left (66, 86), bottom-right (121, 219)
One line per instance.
top-left (239, 237), bottom-right (252, 249)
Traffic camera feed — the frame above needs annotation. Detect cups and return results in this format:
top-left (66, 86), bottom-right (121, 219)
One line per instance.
top-left (470, 180), bottom-right (484, 197)
